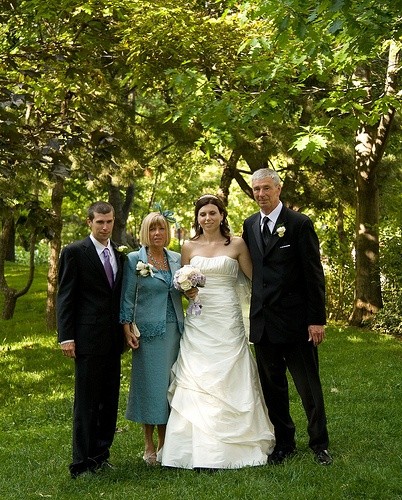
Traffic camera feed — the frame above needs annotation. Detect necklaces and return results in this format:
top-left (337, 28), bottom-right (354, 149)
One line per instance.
top-left (147, 250), bottom-right (167, 270)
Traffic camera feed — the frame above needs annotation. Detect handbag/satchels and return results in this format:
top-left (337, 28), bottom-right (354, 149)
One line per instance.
top-left (130, 323), bottom-right (140, 338)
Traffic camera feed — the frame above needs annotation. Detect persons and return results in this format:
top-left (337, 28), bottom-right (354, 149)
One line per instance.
top-left (161, 193), bottom-right (276, 469)
top-left (120, 212), bottom-right (185, 466)
top-left (242, 168), bottom-right (332, 466)
top-left (56, 201), bottom-right (128, 479)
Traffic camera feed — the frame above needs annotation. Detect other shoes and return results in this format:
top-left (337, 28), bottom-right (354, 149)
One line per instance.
top-left (71, 461), bottom-right (119, 480)
top-left (271, 448), bottom-right (295, 464)
top-left (142, 450), bottom-right (158, 465)
top-left (316, 449), bottom-right (332, 467)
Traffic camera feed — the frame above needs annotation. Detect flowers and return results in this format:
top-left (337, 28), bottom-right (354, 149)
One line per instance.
top-left (173, 265), bottom-right (206, 316)
top-left (275, 222), bottom-right (286, 238)
top-left (116, 245), bottom-right (133, 256)
top-left (136, 261), bottom-right (155, 278)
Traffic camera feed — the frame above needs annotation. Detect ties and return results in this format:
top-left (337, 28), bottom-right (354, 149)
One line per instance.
top-left (103, 248), bottom-right (115, 288)
top-left (261, 217), bottom-right (272, 250)
